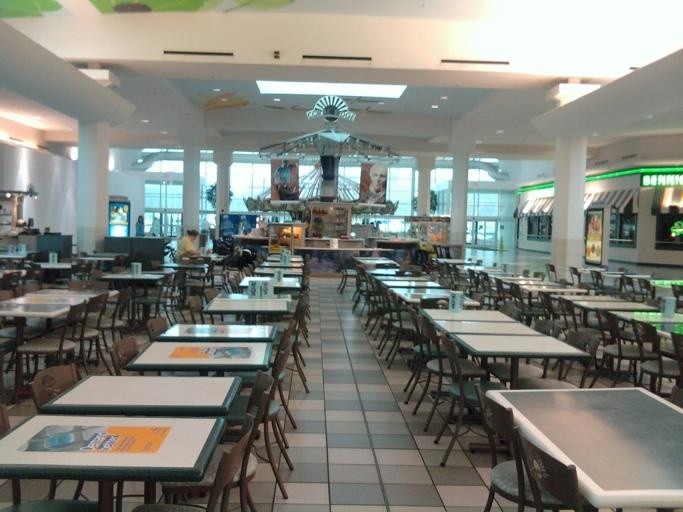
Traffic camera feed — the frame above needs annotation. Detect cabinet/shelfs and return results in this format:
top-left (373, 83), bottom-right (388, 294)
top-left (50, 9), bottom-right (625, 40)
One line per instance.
top-left (305, 203), bottom-right (351, 238)
top-left (0, 193), bottom-right (23, 252)
top-left (268, 223), bottom-right (294, 255)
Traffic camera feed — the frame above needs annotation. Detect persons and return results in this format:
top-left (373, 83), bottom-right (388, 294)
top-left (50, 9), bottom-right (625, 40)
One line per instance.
top-left (135, 215), bottom-right (146, 237)
top-left (175, 229), bottom-right (200, 264)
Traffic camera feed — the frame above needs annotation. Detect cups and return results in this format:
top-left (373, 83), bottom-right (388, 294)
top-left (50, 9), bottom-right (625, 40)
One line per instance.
top-left (131, 262), bottom-right (142, 275)
top-left (660, 297), bottom-right (677, 318)
top-left (286, 251), bottom-right (290, 260)
top-left (283, 254), bottom-right (288, 264)
top-left (280, 252), bottom-right (284, 262)
top-left (282, 250), bottom-right (285, 253)
top-left (8, 245), bottom-right (15, 255)
top-left (49, 253), bottom-right (57, 263)
top-left (274, 270), bottom-right (283, 282)
top-left (18, 244), bottom-right (26, 255)
top-left (449, 291), bottom-right (463, 312)
top-left (249, 280), bottom-right (261, 298)
top-left (262, 280), bottom-right (274, 296)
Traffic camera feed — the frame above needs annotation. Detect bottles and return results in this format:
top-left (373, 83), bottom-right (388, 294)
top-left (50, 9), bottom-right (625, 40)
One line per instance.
top-left (219, 215), bottom-right (236, 250)
top-left (239, 215), bottom-right (252, 235)
